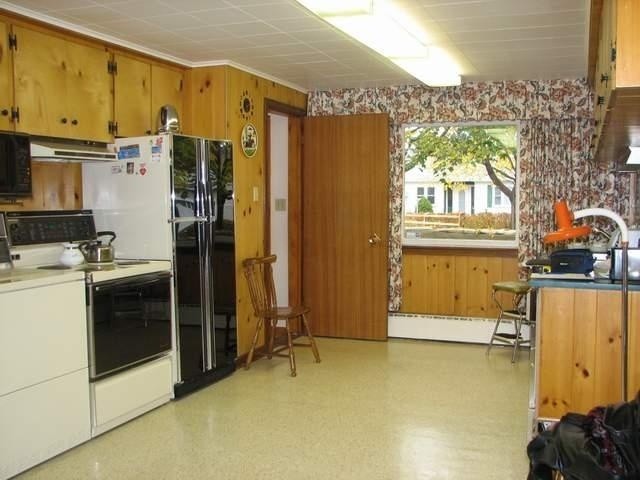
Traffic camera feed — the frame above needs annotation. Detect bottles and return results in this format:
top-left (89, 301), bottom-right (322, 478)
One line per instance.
top-left (60, 243), bottom-right (84, 267)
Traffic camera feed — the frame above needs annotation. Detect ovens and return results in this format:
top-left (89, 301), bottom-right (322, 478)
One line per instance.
top-left (83, 271), bottom-right (176, 382)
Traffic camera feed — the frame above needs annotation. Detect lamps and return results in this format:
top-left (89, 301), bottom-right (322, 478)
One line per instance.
top-left (544, 202), bottom-right (629, 403)
top-left (296, 0), bottom-right (462, 87)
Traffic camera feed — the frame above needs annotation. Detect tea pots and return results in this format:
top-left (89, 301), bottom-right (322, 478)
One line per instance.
top-left (81, 231), bottom-right (116, 263)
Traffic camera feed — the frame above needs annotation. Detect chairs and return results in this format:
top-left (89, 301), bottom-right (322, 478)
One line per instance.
top-left (0, 281), bottom-right (92, 480)
top-left (243, 255), bottom-right (321, 377)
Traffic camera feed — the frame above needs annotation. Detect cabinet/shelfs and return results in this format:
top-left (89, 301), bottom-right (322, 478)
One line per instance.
top-left (586, 0), bottom-right (640, 162)
top-left (89, 356), bottom-right (172, 438)
top-left (0, 21), bottom-right (184, 144)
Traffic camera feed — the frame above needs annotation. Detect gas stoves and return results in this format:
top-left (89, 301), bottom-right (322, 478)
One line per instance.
top-left (31, 255), bottom-right (174, 283)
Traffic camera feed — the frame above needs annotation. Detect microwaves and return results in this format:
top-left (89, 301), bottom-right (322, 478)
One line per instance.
top-left (0, 130), bottom-right (36, 198)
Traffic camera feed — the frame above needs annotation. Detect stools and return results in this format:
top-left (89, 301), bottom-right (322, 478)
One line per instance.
top-left (485, 281), bottom-right (536, 365)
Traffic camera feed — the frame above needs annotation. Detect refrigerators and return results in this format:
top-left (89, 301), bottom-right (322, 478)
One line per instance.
top-left (80, 131), bottom-right (238, 404)
